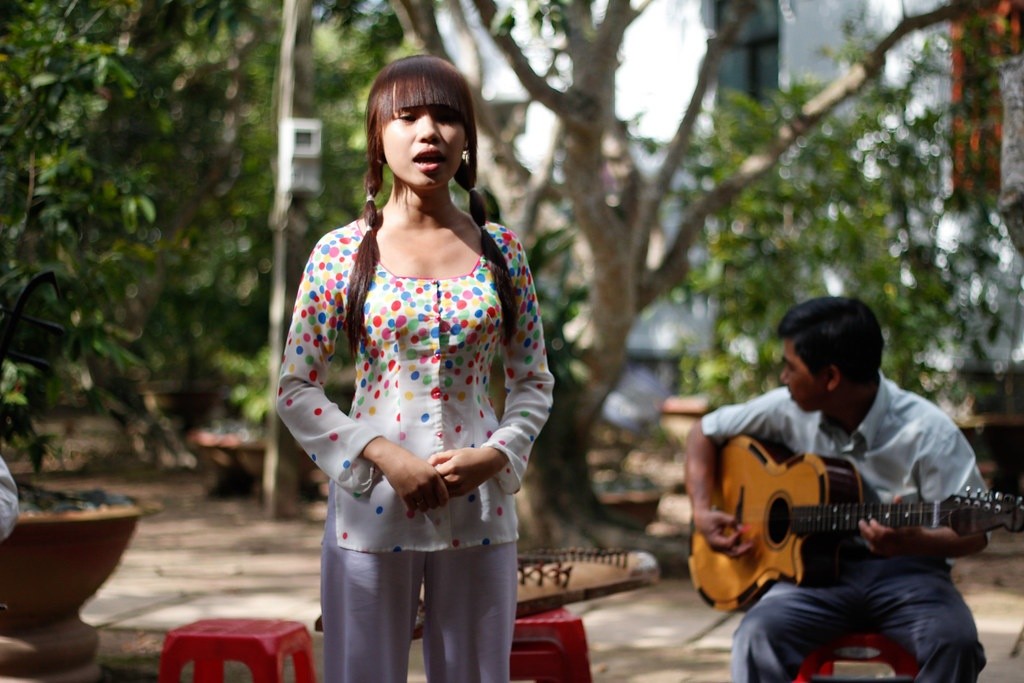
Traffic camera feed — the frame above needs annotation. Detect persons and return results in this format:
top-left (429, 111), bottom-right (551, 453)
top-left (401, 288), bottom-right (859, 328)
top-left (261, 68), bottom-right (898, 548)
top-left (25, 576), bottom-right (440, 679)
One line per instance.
top-left (276, 54), bottom-right (555, 683)
top-left (685, 296), bottom-right (992, 682)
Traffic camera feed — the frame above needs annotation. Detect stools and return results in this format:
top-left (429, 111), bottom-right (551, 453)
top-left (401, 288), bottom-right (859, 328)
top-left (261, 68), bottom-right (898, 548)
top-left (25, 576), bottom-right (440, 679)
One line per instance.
top-left (509, 607), bottom-right (594, 683)
top-left (793, 631), bottom-right (924, 682)
top-left (157, 618), bottom-right (318, 683)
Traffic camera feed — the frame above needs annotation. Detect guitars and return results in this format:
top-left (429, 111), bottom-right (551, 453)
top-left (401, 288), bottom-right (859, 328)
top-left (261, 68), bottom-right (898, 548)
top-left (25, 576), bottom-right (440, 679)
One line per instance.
top-left (688, 434), bottom-right (1024, 612)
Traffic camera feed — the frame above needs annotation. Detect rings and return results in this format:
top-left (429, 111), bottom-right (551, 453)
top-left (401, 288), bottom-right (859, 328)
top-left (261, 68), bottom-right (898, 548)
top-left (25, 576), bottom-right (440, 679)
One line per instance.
top-left (416, 498), bottom-right (424, 505)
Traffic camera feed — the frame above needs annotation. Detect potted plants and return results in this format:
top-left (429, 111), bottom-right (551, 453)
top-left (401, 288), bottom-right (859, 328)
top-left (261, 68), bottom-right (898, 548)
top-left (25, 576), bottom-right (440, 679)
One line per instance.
top-left (1, 1), bottom-right (236, 633)
top-left (0, 477), bottom-right (139, 668)
